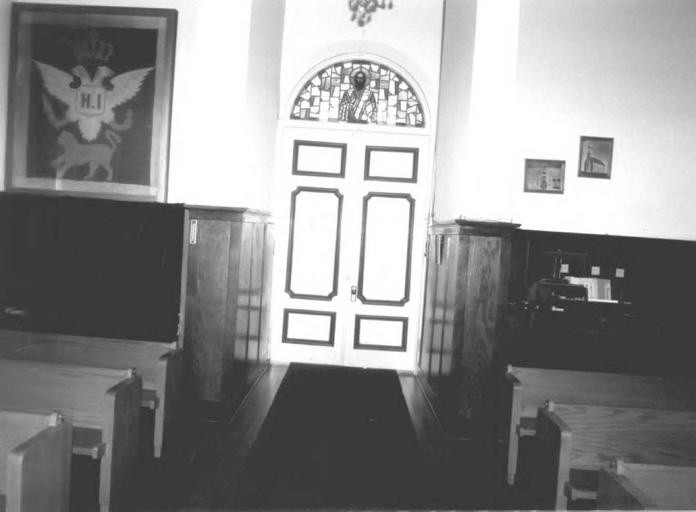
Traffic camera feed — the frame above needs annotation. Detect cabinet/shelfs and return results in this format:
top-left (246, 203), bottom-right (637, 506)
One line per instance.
top-left (183, 207), bottom-right (274, 421)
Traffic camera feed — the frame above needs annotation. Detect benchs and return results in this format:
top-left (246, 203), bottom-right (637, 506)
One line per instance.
top-left (2, 409), bottom-right (73, 512)
top-left (1, 359), bottom-right (142, 512)
top-left (500, 365), bottom-right (695, 484)
top-left (536, 400), bottom-right (696, 511)
top-left (598, 460), bottom-right (696, 512)
top-left (0, 328), bottom-right (178, 456)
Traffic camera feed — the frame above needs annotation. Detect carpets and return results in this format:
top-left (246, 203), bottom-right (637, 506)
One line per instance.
top-left (230, 362), bottom-right (440, 511)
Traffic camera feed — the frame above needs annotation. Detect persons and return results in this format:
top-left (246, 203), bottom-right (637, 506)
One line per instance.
top-left (338, 71), bottom-right (377, 124)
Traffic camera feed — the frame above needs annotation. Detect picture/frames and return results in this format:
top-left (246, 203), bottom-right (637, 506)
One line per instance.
top-left (578, 136), bottom-right (615, 179)
top-left (4, 2), bottom-right (178, 203)
top-left (523, 158), bottom-right (565, 195)
